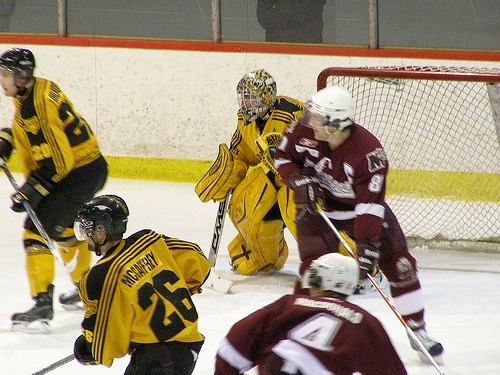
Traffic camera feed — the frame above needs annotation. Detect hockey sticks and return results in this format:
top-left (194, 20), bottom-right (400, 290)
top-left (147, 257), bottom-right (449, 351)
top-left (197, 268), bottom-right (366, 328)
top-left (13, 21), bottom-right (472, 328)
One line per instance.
top-left (202, 186), bottom-right (234, 295)
top-left (33, 352), bottom-right (89, 375)
top-left (314, 200), bottom-right (449, 375)
top-left (0, 158), bottom-right (82, 304)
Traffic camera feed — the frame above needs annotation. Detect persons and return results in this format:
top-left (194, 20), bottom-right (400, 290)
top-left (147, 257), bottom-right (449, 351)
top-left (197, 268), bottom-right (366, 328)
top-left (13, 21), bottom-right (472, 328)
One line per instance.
top-left (257, 0), bottom-right (326, 44)
top-left (73, 195), bottom-right (211, 375)
top-left (272, 84), bottom-right (445, 365)
top-left (0, 48), bottom-right (112, 329)
top-left (214, 252), bottom-right (408, 375)
top-left (194, 69), bottom-right (387, 295)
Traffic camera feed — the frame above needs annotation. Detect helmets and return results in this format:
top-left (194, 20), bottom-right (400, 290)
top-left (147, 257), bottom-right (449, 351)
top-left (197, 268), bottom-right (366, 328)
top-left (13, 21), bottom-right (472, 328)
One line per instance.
top-left (301, 252), bottom-right (360, 296)
top-left (236, 69), bottom-right (277, 126)
top-left (0, 48), bottom-right (35, 88)
top-left (76, 195), bottom-right (129, 237)
top-left (306, 86), bottom-right (356, 136)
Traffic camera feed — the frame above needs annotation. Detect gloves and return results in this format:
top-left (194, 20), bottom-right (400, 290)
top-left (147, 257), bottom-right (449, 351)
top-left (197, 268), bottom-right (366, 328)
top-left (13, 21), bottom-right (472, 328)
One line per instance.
top-left (74, 335), bottom-right (98, 365)
top-left (10, 169), bottom-right (58, 212)
top-left (355, 240), bottom-right (381, 281)
top-left (287, 166), bottom-right (328, 223)
top-left (0, 127), bottom-right (15, 168)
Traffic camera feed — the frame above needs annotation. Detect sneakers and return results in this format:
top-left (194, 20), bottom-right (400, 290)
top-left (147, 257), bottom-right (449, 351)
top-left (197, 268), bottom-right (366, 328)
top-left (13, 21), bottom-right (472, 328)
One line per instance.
top-left (59, 282), bottom-right (84, 311)
top-left (11, 284), bottom-right (54, 335)
top-left (408, 320), bottom-right (445, 366)
